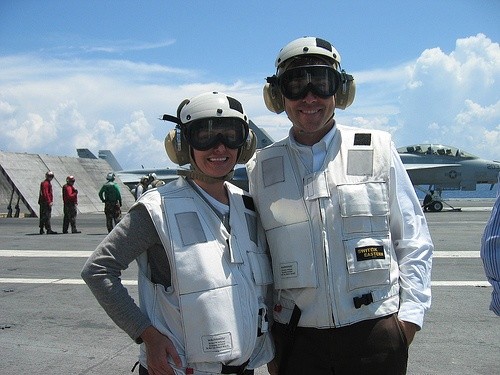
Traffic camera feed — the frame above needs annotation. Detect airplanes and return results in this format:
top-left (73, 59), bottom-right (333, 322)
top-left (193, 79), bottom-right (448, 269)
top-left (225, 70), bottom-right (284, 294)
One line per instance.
top-left (75, 120), bottom-right (500, 212)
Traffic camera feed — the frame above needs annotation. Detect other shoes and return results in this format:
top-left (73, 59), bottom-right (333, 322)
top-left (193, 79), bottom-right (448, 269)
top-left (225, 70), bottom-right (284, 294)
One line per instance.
top-left (47, 230), bottom-right (57, 234)
top-left (63, 231), bottom-right (68, 234)
top-left (40, 229), bottom-right (45, 234)
top-left (72, 230), bottom-right (81, 233)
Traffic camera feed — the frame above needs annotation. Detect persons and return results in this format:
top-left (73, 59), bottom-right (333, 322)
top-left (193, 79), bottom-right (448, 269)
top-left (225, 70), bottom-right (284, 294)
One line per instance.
top-left (62, 175), bottom-right (81, 234)
top-left (480, 192), bottom-right (500, 316)
top-left (244, 36), bottom-right (434, 375)
top-left (135, 172), bottom-right (161, 202)
top-left (38, 171), bottom-right (57, 234)
top-left (81, 90), bottom-right (274, 375)
top-left (98, 172), bottom-right (122, 234)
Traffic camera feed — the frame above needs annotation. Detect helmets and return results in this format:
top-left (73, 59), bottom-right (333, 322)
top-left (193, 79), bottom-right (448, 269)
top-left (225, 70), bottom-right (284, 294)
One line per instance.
top-left (45, 171), bottom-right (54, 179)
top-left (66, 176), bottom-right (75, 183)
top-left (274, 36), bottom-right (341, 69)
top-left (141, 175), bottom-right (149, 183)
top-left (148, 173), bottom-right (157, 180)
top-left (106, 173), bottom-right (115, 181)
top-left (178, 91), bottom-right (250, 130)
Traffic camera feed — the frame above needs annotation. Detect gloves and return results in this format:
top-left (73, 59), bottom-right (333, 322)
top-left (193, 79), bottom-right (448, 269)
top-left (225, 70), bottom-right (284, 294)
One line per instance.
top-left (74, 189), bottom-right (78, 193)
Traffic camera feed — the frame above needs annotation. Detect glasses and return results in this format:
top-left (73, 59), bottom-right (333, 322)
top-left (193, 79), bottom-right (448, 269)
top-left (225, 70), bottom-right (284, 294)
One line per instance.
top-left (277, 63), bottom-right (346, 102)
top-left (182, 116), bottom-right (250, 151)
